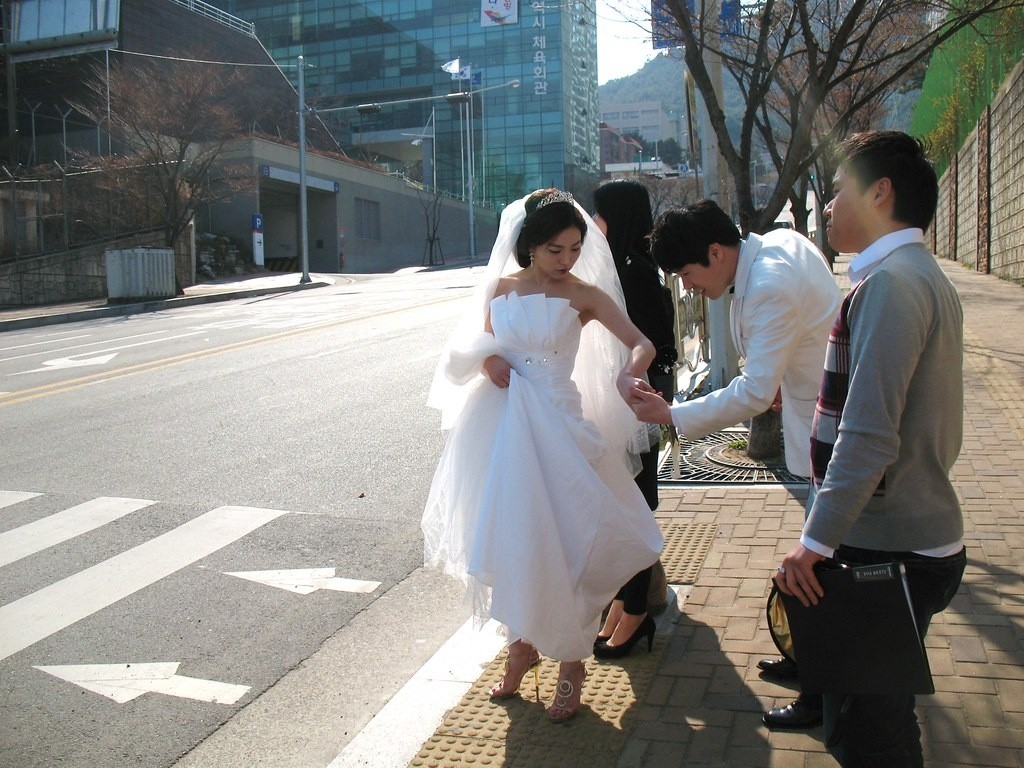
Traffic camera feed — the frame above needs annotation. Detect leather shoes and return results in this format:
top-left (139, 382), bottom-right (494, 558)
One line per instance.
top-left (761, 701), bottom-right (823, 729)
top-left (756, 656), bottom-right (799, 672)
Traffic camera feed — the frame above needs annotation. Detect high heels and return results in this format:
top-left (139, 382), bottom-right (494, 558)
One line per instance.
top-left (548, 662), bottom-right (587, 723)
top-left (489, 647), bottom-right (542, 699)
top-left (593, 613), bottom-right (656, 659)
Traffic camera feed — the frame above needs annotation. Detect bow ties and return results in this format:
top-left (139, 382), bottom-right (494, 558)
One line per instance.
top-left (729, 286), bottom-right (734, 294)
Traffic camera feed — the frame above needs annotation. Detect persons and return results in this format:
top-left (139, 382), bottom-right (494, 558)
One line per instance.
top-left (628, 198), bottom-right (844, 731)
top-left (772, 127), bottom-right (968, 768)
top-left (592, 180), bottom-right (679, 662)
top-left (420, 186), bottom-right (665, 722)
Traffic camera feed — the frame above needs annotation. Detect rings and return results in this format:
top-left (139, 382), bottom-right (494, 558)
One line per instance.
top-left (777, 567), bottom-right (785, 573)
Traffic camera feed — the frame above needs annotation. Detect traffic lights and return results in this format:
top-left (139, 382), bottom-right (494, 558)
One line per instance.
top-left (810, 175), bottom-right (814, 180)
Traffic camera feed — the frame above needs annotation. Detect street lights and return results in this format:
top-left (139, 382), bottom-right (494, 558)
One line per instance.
top-left (461, 79), bottom-right (520, 259)
top-left (750, 159), bottom-right (757, 211)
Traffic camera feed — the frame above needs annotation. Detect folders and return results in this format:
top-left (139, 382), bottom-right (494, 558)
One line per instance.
top-left (771, 561), bottom-right (936, 695)
